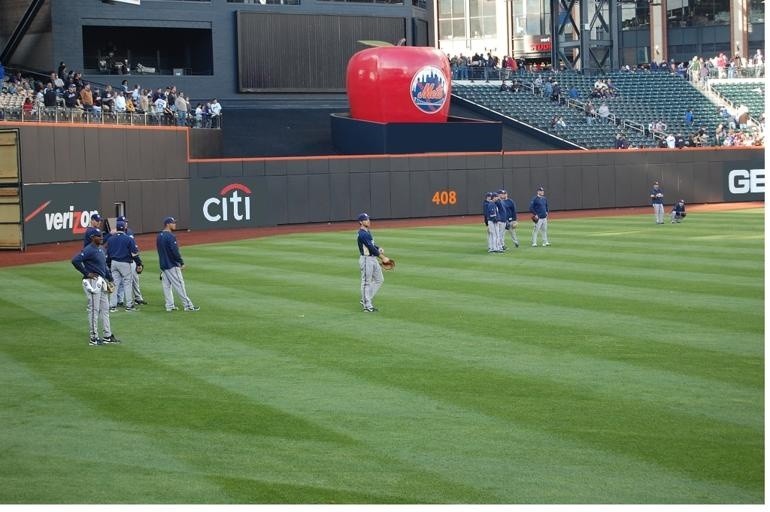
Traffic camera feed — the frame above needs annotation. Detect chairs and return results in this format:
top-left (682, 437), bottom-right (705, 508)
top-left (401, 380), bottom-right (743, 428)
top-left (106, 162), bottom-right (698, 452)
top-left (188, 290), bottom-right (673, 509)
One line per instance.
top-left (0, 81), bottom-right (26, 110)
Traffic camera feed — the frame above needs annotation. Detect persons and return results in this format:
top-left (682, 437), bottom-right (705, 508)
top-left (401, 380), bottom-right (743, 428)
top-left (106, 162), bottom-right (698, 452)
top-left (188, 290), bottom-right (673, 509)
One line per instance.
top-left (622, 50), bottom-right (765, 78)
top-left (552, 116), bottom-right (567, 128)
top-left (1, 49), bottom-right (225, 130)
top-left (669, 200), bottom-right (686, 223)
top-left (586, 102), bottom-right (611, 122)
top-left (482, 187), bottom-right (551, 251)
top-left (71, 214), bottom-right (201, 346)
top-left (650, 182), bottom-right (665, 224)
top-left (354, 212), bottom-right (396, 314)
top-left (614, 134), bottom-right (631, 149)
top-left (501, 75), bottom-right (614, 101)
top-left (447, 51), bottom-right (555, 73)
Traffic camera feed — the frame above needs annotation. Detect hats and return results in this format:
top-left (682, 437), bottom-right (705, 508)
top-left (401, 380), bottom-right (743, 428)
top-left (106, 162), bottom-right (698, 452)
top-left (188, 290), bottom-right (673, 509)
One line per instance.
top-left (485, 189), bottom-right (508, 200)
top-left (91, 214), bottom-right (103, 222)
top-left (115, 216), bottom-right (130, 228)
top-left (537, 186), bottom-right (546, 192)
top-left (358, 213), bottom-right (370, 221)
top-left (89, 228), bottom-right (103, 237)
top-left (164, 217), bottom-right (179, 224)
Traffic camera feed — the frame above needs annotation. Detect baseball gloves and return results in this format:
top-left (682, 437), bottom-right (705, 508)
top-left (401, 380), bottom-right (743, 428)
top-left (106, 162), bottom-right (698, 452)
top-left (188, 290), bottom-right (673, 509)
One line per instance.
top-left (381, 257), bottom-right (395, 271)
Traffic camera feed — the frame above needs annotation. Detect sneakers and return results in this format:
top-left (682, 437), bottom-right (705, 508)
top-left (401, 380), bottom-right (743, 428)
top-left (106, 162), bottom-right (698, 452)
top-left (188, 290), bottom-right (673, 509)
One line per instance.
top-left (88, 337), bottom-right (103, 348)
top-left (101, 334), bottom-right (121, 344)
top-left (166, 306), bottom-right (181, 312)
top-left (135, 299), bottom-right (148, 305)
top-left (359, 297), bottom-right (378, 313)
top-left (110, 302), bottom-right (124, 313)
top-left (125, 305), bottom-right (142, 313)
top-left (182, 304), bottom-right (200, 312)
